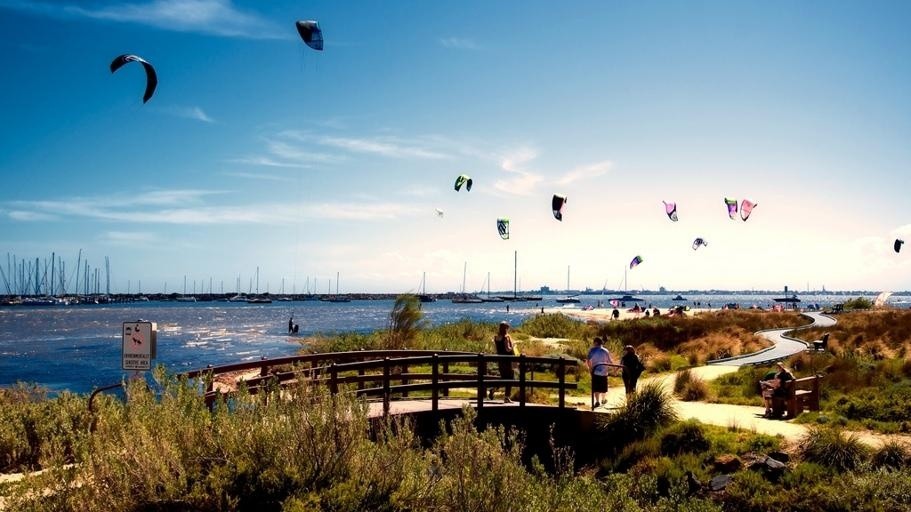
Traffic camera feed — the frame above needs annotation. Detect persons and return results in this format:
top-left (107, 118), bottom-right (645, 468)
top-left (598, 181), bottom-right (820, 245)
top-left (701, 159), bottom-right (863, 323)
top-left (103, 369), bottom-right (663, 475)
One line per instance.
top-left (763, 361), bottom-right (797, 420)
top-left (288, 317), bottom-right (295, 333)
top-left (489, 320), bottom-right (518, 403)
top-left (616, 345), bottom-right (646, 407)
top-left (612, 306), bottom-right (620, 321)
top-left (587, 337), bottom-right (618, 407)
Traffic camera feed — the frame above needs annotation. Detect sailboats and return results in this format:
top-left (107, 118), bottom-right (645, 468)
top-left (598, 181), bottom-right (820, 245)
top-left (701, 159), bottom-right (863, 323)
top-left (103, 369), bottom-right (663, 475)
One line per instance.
top-left (414, 271), bottom-right (434, 302)
top-left (451, 252), bottom-right (542, 303)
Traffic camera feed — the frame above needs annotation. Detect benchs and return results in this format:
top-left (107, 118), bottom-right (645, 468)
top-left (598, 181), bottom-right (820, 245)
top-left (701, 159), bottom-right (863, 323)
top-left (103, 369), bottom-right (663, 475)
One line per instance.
top-left (832, 304), bottom-right (844, 313)
top-left (764, 374), bottom-right (823, 417)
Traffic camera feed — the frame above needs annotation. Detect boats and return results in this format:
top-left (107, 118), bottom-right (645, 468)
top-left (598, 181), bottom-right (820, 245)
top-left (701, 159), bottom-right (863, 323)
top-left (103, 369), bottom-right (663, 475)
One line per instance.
top-left (608, 296), bottom-right (642, 301)
top-left (555, 296), bottom-right (579, 303)
top-left (672, 296), bottom-right (686, 300)
top-left (773, 298), bottom-right (800, 302)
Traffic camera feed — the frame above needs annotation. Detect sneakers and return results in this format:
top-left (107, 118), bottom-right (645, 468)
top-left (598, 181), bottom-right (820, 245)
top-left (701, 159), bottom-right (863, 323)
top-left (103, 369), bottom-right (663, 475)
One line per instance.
top-left (594, 399), bottom-right (608, 407)
top-left (505, 398), bottom-right (512, 403)
top-left (489, 389), bottom-right (494, 400)
